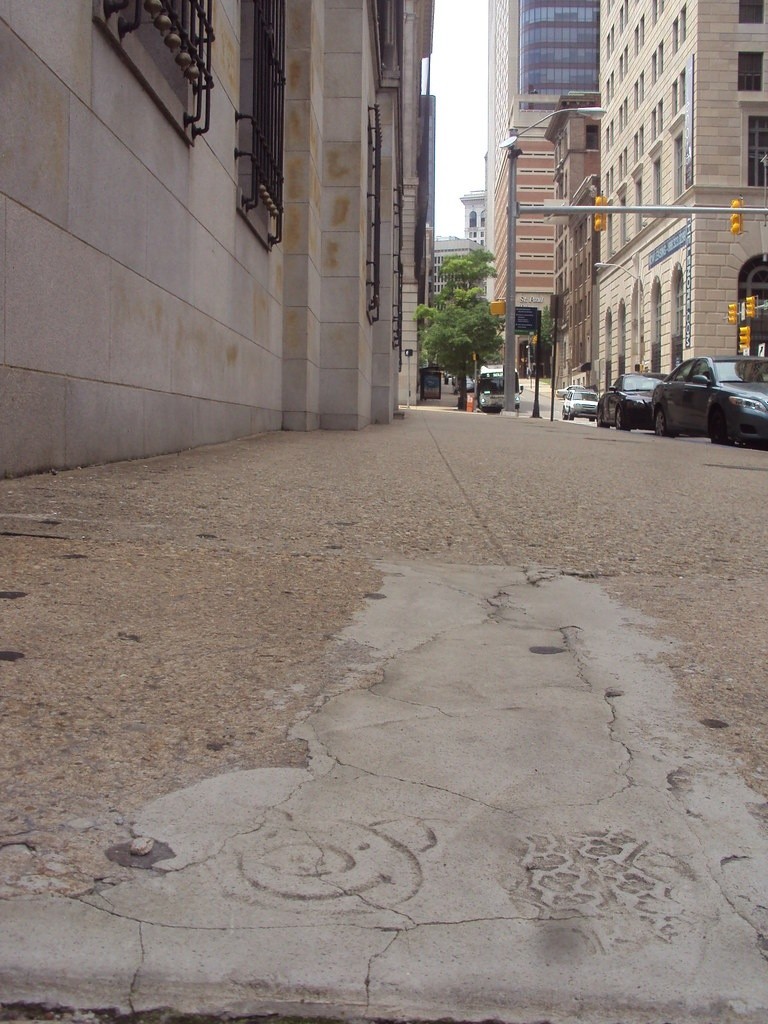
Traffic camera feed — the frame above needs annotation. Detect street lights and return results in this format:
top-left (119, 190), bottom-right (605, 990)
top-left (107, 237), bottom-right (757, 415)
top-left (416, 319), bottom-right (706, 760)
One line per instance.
top-left (594, 262), bottom-right (643, 373)
top-left (497, 105), bottom-right (608, 418)
top-left (739, 326), bottom-right (751, 353)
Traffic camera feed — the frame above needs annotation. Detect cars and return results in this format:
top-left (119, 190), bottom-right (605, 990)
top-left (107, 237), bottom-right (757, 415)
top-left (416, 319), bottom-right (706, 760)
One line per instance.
top-left (562, 388), bottom-right (599, 422)
top-left (555, 384), bottom-right (586, 400)
top-left (443, 371), bottom-right (475, 393)
top-left (650, 354), bottom-right (768, 451)
top-left (595, 372), bottom-right (670, 431)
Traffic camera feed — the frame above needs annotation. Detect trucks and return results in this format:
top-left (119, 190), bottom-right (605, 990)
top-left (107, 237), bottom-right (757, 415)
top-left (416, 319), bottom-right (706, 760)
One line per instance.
top-left (478, 364), bottom-right (524, 414)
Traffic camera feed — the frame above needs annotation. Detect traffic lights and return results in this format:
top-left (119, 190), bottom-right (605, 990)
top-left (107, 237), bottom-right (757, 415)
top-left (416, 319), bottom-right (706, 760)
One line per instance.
top-left (728, 304), bottom-right (737, 325)
top-left (745, 296), bottom-right (756, 318)
